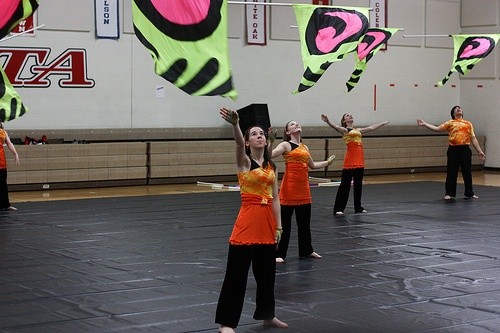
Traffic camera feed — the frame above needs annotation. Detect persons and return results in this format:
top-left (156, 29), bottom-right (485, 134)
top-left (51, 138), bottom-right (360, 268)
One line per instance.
top-left (0, 122), bottom-right (20, 210)
top-left (417, 106), bottom-right (486, 200)
top-left (320, 113), bottom-right (389, 215)
top-left (215, 107), bottom-right (288, 333)
top-left (267, 120), bottom-right (336, 263)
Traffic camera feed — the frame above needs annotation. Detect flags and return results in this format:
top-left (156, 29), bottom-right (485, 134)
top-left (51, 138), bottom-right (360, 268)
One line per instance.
top-left (0, 0), bottom-right (39, 122)
top-left (290, 4), bottom-right (369, 95)
top-left (440, 34), bottom-right (500, 87)
top-left (132, 0), bottom-right (237, 100)
top-left (345, 27), bottom-right (399, 92)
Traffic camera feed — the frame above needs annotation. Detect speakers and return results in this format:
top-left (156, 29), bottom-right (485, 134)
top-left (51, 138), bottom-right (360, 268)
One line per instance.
top-left (237, 103), bottom-right (271, 136)
